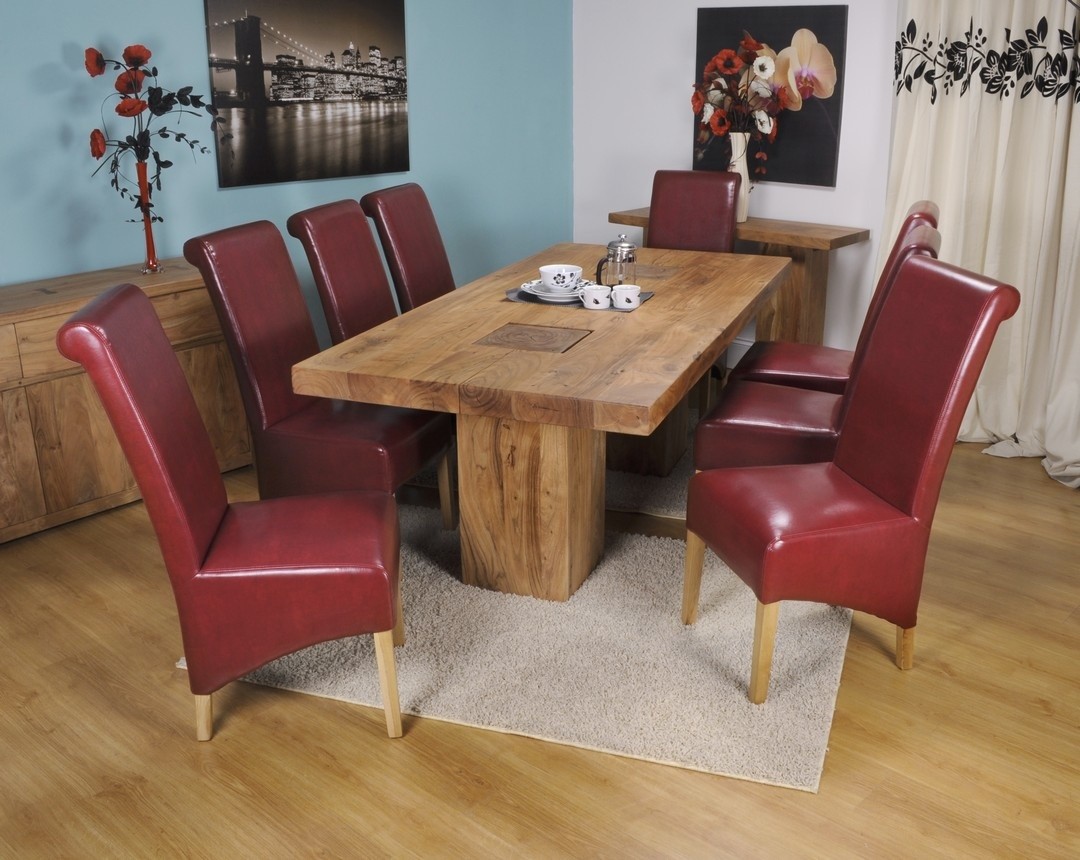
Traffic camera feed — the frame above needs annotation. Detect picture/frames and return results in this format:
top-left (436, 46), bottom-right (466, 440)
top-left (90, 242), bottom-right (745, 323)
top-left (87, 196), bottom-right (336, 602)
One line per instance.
top-left (204, 0), bottom-right (411, 187)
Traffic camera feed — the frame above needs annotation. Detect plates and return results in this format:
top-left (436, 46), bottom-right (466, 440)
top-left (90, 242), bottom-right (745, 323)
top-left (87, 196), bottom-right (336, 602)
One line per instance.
top-left (521, 280), bottom-right (595, 303)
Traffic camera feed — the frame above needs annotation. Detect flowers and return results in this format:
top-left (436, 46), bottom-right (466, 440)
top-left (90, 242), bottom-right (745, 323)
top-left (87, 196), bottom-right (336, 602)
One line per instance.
top-left (82, 45), bottom-right (237, 232)
top-left (688, 30), bottom-right (788, 194)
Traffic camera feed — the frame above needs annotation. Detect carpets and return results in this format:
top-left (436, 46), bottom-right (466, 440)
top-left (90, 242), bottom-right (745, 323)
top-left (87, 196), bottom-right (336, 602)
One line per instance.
top-left (407, 407), bottom-right (696, 520)
top-left (172, 501), bottom-right (857, 795)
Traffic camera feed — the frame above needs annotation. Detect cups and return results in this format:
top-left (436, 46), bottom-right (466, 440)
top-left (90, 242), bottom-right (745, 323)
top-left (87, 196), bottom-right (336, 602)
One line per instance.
top-left (579, 285), bottom-right (611, 310)
top-left (611, 285), bottom-right (643, 308)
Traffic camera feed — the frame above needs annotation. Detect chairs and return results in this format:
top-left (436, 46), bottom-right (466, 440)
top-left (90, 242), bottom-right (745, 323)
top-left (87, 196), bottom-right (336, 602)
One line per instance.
top-left (725, 199), bottom-right (942, 395)
top-left (360, 182), bottom-right (458, 313)
top-left (676, 253), bottom-right (1022, 706)
top-left (56, 282), bottom-right (406, 742)
top-left (688, 225), bottom-right (942, 475)
top-left (182, 218), bottom-right (456, 532)
top-left (287, 198), bottom-right (401, 345)
top-left (646, 168), bottom-right (743, 253)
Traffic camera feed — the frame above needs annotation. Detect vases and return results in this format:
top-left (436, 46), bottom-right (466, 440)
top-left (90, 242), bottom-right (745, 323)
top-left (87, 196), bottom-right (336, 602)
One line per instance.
top-left (727, 132), bottom-right (752, 222)
top-left (135, 162), bottom-right (165, 275)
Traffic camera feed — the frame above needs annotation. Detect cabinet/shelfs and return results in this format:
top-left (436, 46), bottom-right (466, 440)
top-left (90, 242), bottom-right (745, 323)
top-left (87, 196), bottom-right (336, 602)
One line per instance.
top-left (0, 284), bottom-right (48, 544)
top-left (14, 255), bottom-right (257, 526)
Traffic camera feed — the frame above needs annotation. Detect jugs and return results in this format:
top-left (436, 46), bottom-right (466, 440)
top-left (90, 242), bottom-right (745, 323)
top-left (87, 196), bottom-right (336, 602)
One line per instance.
top-left (597, 233), bottom-right (638, 287)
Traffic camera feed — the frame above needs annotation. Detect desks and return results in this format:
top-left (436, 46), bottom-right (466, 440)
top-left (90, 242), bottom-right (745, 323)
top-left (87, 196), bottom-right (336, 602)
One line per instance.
top-left (608, 204), bottom-right (870, 343)
top-left (290, 240), bottom-right (794, 601)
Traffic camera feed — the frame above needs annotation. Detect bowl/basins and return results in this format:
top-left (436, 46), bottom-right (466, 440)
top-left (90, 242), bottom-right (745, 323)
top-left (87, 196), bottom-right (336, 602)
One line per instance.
top-left (538, 264), bottom-right (583, 293)
